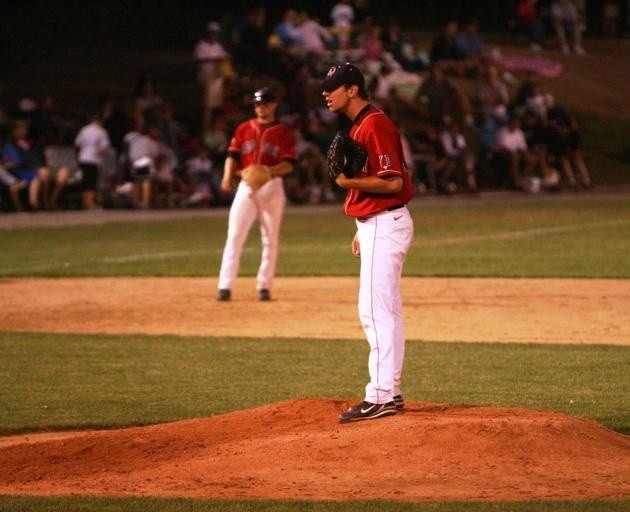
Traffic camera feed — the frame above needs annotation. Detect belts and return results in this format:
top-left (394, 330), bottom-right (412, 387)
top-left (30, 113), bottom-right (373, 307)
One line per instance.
top-left (388, 204), bottom-right (404, 211)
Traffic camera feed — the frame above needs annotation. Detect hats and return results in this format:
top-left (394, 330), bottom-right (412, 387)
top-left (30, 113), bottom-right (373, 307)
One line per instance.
top-left (319, 62), bottom-right (364, 92)
top-left (251, 87), bottom-right (276, 104)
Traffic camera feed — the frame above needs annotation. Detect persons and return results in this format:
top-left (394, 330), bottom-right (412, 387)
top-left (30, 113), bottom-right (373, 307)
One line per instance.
top-left (316, 62), bottom-right (415, 423)
top-left (217, 87), bottom-right (298, 301)
top-left (0, 0), bottom-right (619, 214)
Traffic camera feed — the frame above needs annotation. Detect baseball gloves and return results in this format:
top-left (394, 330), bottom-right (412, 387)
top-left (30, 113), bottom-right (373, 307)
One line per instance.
top-left (240, 164), bottom-right (272, 190)
top-left (327, 131), bottom-right (366, 180)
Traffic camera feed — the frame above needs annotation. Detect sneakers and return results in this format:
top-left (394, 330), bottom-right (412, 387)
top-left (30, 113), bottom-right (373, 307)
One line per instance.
top-left (338, 401), bottom-right (397, 421)
top-left (260, 289), bottom-right (270, 300)
top-left (393, 395), bottom-right (404, 408)
top-left (217, 290), bottom-right (230, 300)
